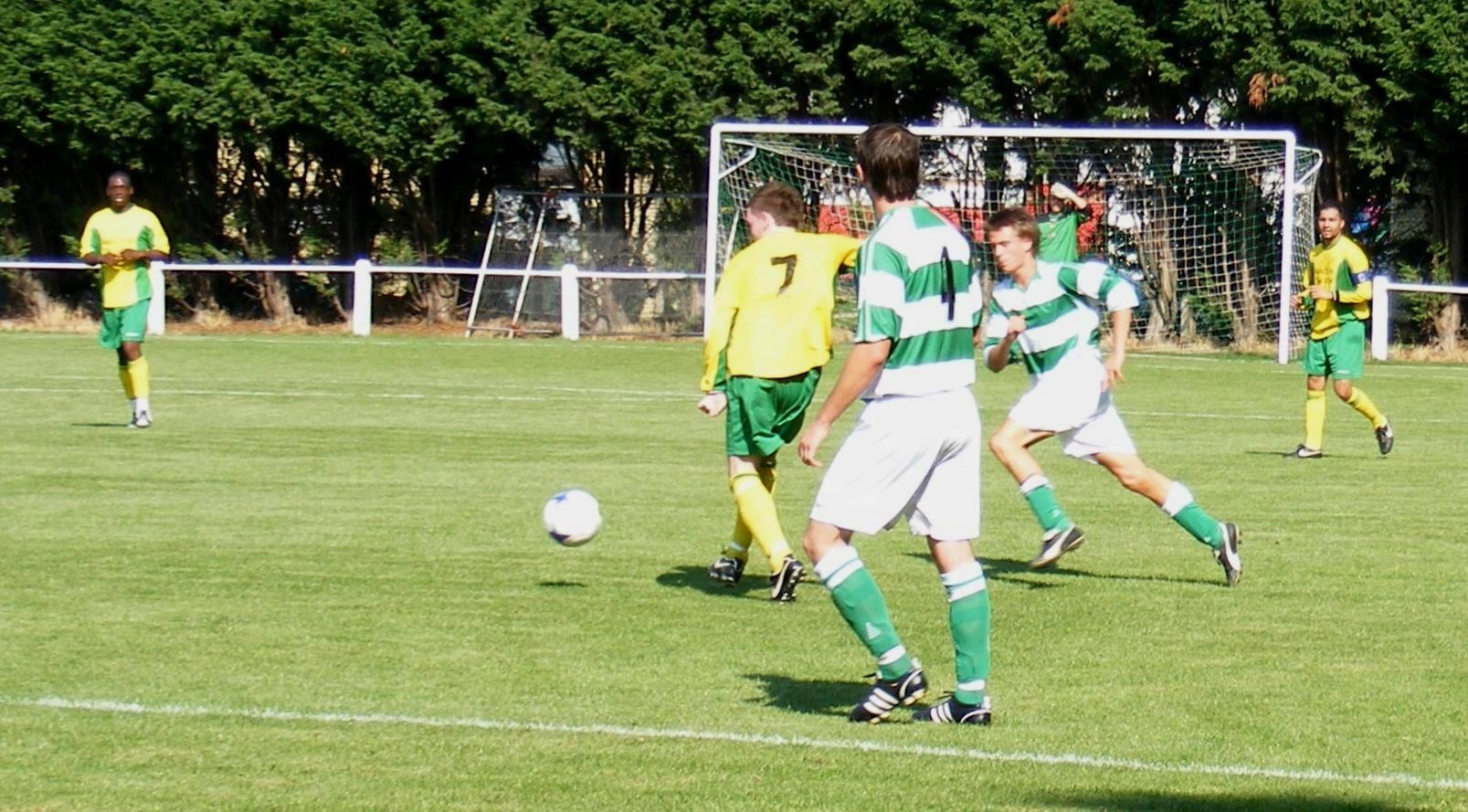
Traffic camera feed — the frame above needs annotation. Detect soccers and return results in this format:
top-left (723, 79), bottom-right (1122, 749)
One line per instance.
top-left (543, 489), bottom-right (601, 548)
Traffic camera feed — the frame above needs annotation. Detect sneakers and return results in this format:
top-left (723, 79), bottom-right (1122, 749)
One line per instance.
top-left (849, 667), bottom-right (928, 725)
top-left (911, 691), bottom-right (991, 726)
top-left (1283, 444), bottom-right (1322, 460)
top-left (1375, 415), bottom-right (1394, 455)
top-left (1211, 522), bottom-right (1243, 587)
top-left (770, 555), bottom-right (808, 600)
top-left (1029, 524), bottom-right (1087, 569)
top-left (708, 554), bottom-right (746, 587)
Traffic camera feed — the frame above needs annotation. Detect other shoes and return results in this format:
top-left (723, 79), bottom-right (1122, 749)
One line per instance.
top-left (129, 409), bottom-right (153, 428)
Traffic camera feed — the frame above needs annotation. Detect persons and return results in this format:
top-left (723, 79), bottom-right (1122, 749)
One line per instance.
top-left (1287, 202), bottom-right (1393, 458)
top-left (977, 204), bottom-right (1245, 589)
top-left (699, 179), bottom-right (867, 599)
top-left (796, 122), bottom-right (991, 728)
top-left (80, 172), bottom-right (170, 429)
